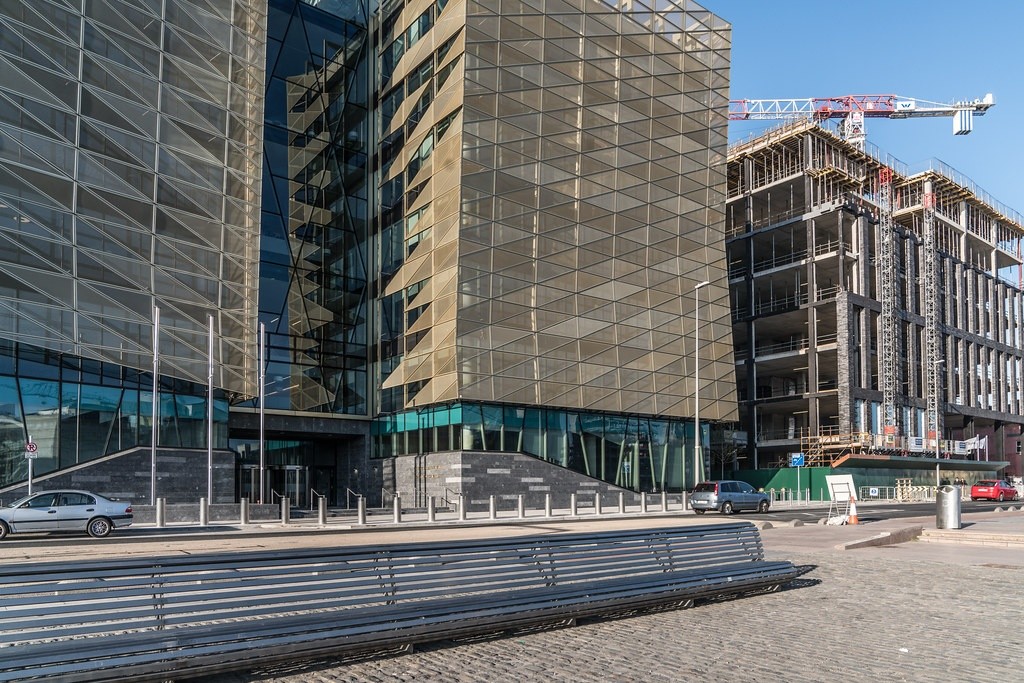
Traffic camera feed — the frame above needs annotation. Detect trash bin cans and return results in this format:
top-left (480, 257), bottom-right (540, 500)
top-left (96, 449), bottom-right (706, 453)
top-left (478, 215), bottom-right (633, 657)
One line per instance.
top-left (936, 484), bottom-right (961, 530)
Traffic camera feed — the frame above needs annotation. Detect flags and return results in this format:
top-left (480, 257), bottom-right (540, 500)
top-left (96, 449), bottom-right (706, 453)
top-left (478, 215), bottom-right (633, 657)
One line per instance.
top-left (965, 437), bottom-right (978, 449)
top-left (979, 437), bottom-right (985, 449)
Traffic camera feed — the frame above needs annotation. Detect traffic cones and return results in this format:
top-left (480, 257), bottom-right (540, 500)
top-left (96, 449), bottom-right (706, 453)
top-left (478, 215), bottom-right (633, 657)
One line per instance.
top-left (848, 496), bottom-right (861, 526)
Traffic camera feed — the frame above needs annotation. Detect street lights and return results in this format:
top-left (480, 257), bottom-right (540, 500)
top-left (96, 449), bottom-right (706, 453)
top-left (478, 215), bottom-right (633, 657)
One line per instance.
top-left (934, 360), bottom-right (946, 485)
top-left (694, 281), bottom-right (713, 486)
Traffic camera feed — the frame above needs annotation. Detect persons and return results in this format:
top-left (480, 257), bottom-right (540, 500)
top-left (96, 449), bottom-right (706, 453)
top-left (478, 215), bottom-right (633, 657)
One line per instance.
top-left (954, 477), bottom-right (969, 485)
top-left (942, 477), bottom-right (950, 485)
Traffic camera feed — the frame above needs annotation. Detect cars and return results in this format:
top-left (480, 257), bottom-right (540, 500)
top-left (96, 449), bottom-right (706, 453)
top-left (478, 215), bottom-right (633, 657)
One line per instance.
top-left (690, 481), bottom-right (771, 515)
top-left (970, 479), bottom-right (1019, 502)
top-left (0, 489), bottom-right (133, 541)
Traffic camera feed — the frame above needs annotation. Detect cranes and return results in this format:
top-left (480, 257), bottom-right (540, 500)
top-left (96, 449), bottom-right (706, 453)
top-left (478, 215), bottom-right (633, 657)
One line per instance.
top-left (728, 92), bottom-right (995, 144)
